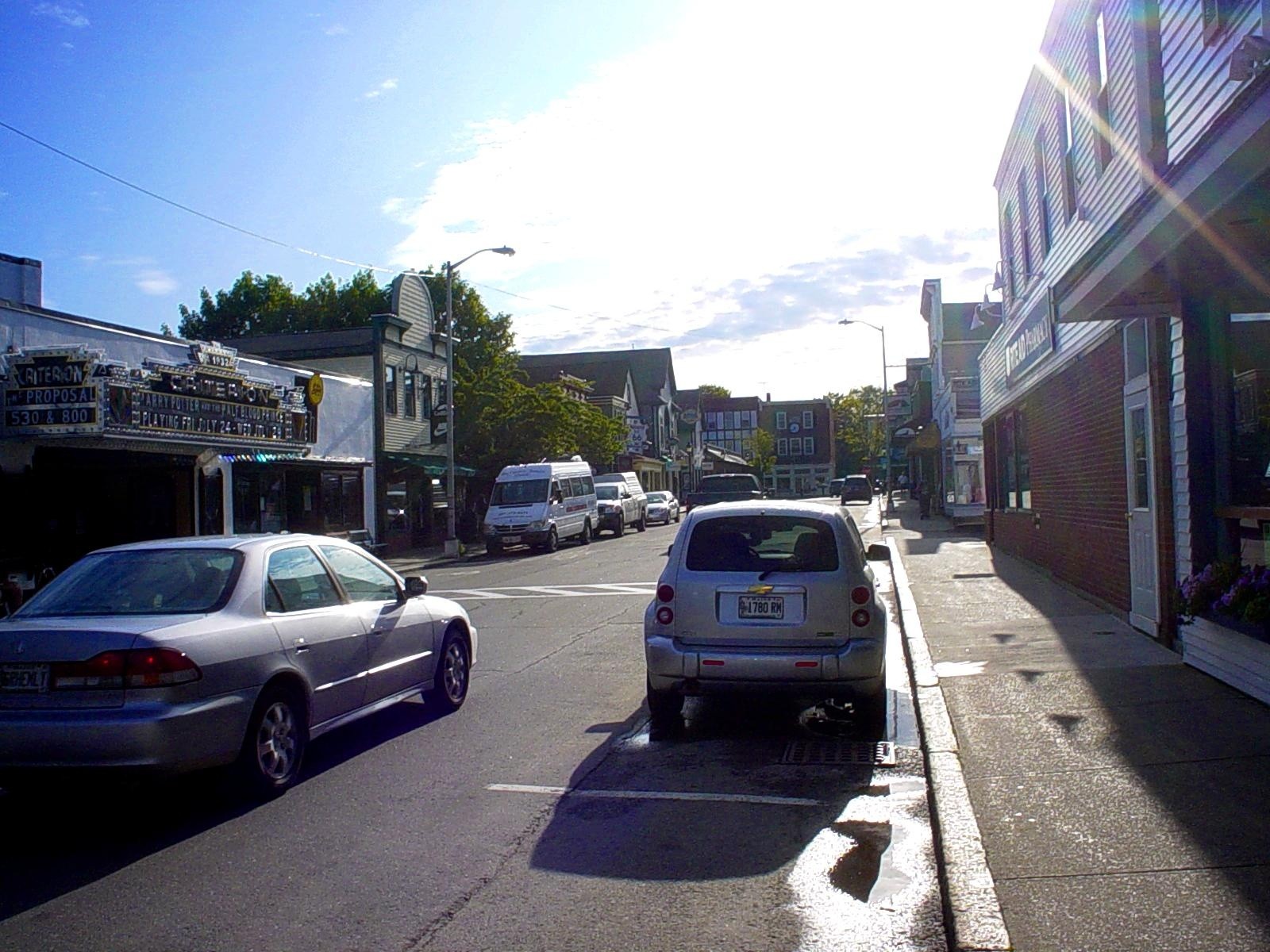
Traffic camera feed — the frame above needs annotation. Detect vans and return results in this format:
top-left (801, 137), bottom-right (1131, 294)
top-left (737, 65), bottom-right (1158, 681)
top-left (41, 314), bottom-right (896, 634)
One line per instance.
top-left (479, 455), bottom-right (601, 551)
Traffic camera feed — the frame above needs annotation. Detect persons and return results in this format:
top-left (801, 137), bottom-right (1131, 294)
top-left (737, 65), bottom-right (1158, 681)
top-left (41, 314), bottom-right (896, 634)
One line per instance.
top-left (915, 476), bottom-right (934, 521)
top-left (898, 471), bottom-right (908, 499)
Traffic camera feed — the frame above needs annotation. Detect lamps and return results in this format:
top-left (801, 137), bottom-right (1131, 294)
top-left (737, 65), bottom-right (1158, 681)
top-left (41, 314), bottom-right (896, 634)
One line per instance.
top-left (399, 353), bottom-right (422, 375)
top-left (1229, 34), bottom-right (1270, 82)
top-left (971, 260), bottom-right (1044, 329)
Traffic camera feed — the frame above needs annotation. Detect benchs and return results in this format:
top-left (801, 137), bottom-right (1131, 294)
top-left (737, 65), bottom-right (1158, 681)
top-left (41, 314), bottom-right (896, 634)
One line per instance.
top-left (326, 529), bottom-right (388, 560)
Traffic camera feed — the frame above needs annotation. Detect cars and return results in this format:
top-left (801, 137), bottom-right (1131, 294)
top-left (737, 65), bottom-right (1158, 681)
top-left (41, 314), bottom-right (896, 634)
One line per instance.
top-left (2, 531), bottom-right (482, 792)
top-left (643, 492), bottom-right (681, 524)
top-left (828, 477), bottom-right (844, 496)
top-left (840, 474), bottom-right (872, 504)
top-left (642, 500), bottom-right (897, 740)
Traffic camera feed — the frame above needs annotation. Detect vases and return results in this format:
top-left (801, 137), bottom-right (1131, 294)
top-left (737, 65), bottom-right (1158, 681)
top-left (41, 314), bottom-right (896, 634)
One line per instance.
top-left (1180, 612), bottom-right (1270, 706)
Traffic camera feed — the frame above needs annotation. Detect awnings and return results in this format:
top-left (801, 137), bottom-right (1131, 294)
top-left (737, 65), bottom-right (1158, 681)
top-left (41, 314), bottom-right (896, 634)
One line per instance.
top-left (904, 422), bottom-right (941, 460)
top-left (385, 455), bottom-right (476, 477)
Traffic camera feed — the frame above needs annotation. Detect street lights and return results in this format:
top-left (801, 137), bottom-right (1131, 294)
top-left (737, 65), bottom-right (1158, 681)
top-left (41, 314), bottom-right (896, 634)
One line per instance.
top-left (443, 243), bottom-right (517, 558)
top-left (839, 317), bottom-right (900, 519)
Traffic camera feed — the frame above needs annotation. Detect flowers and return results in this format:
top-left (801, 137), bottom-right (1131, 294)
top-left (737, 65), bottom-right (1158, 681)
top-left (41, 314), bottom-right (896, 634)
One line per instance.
top-left (1168, 555), bottom-right (1270, 638)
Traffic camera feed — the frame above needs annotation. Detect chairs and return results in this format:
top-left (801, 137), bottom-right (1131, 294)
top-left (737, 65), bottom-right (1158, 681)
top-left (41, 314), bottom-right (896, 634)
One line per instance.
top-left (710, 532), bottom-right (758, 570)
top-left (269, 578), bottom-right (311, 613)
top-left (780, 533), bottom-right (836, 571)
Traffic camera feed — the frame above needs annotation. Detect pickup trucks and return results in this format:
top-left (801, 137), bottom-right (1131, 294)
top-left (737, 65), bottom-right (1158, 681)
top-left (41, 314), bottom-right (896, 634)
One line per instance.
top-left (591, 471), bottom-right (648, 535)
top-left (684, 473), bottom-right (770, 516)
